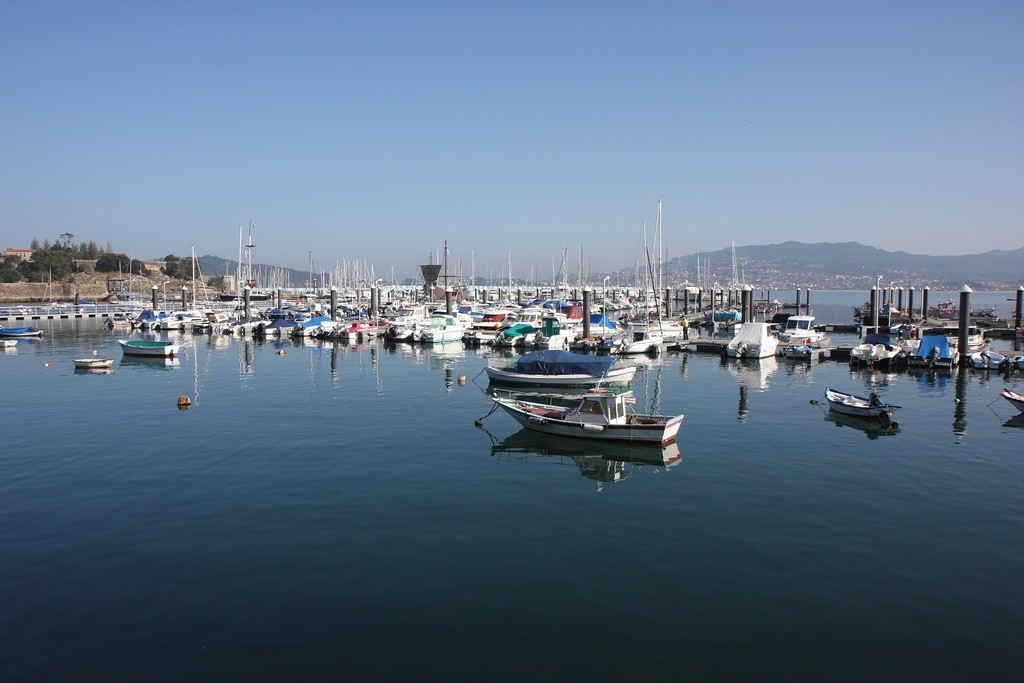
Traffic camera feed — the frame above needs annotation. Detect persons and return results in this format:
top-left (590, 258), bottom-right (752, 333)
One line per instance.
top-left (724, 316), bottom-right (731, 335)
top-left (681, 316), bottom-right (689, 340)
top-left (532, 328), bottom-right (543, 350)
top-left (898, 322), bottom-right (917, 341)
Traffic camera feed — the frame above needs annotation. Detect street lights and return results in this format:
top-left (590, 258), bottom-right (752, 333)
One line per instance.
top-left (876, 275), bottom-right (883, 333)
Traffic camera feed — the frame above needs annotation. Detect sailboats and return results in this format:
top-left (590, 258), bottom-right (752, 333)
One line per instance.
top-left (104, 194), bottom-right (749, 356)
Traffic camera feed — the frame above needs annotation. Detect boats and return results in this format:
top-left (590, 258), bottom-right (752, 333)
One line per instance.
top-left (491, 427), bottom-right (684, 493)
top-left (786, 345), bottom-right (812, 359)
top-left (726, 356), bottom-right (776, 393)
top-left (779, 315), bottom-right (827, 344)
top-left (74, 368), bottom-right (115, 375)
top-left (823, 407), bottom-right (900, 442)
top-left (490, 395), bottom-right (686, 446)
top-left (824, 386), bottom-right (901, 418)
top-left (1000, 413), bottom-right (1024, 430)
top-left (783, 301), bottom-right (807, 309)
top-left (848, 334), bottom-right (1024, 376)
top-left (1, 327), bottom-right (44, 337)
top-left (72, 358), bottom-right (114, 368)
top-left (0, 339), bottom-right (18, 348)
top-left (999, 389), bottom-right (1024, 412)
top-left (482, 352), bottom-right (637, 393)
top-left (118, 356), bottom-right (180, 369)
top-left (726, 322), bottom-right (780, 359)
top-left (0, 348), bottom-right (19, 356)
